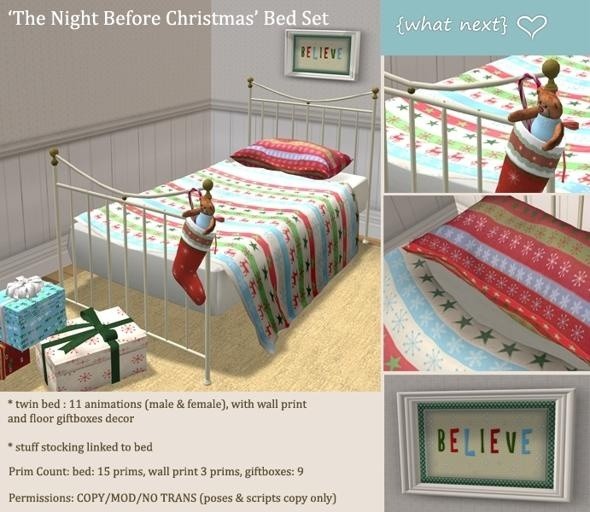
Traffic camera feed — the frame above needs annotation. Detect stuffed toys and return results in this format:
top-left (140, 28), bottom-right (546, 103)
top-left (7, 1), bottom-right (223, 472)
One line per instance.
top-left (507, 87), bottom-right (566, 151)
top-left (181, 196), bottom-right (217, 235)
